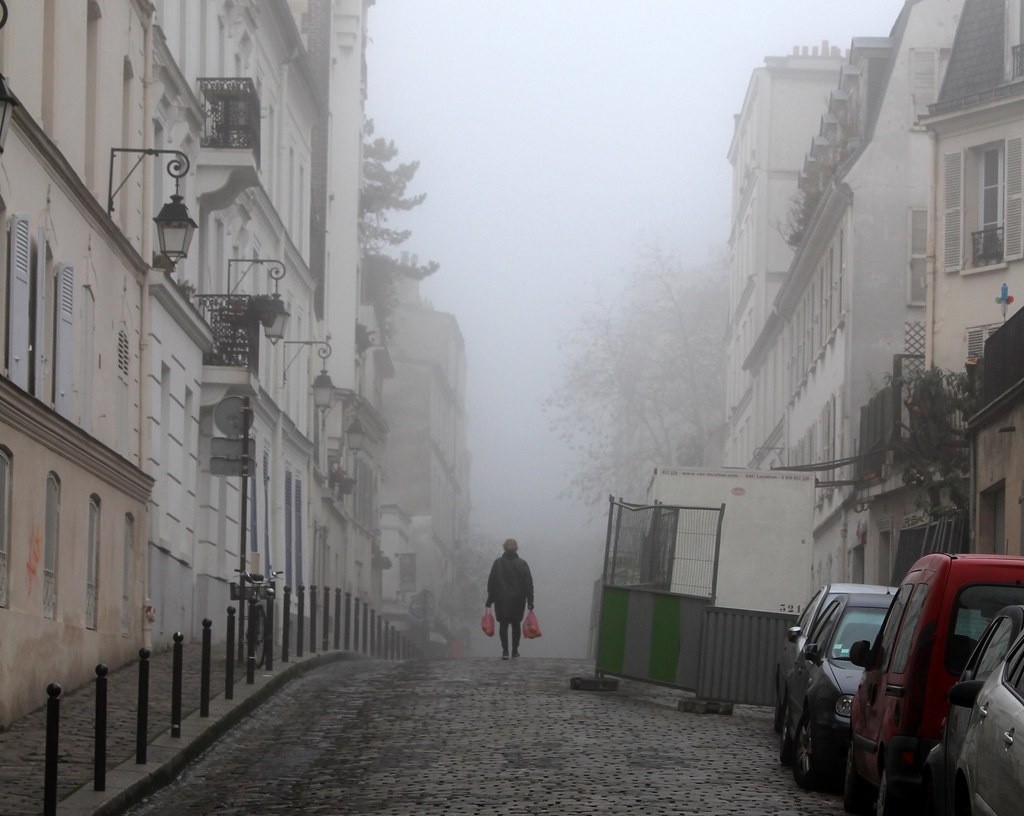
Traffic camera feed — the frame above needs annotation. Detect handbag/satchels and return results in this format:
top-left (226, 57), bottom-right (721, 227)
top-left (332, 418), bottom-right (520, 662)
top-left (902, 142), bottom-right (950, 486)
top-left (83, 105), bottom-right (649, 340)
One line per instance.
top-left (523, 609), bottom-right (542, 639)
top-left (481, 607), bottom-right (494, 637)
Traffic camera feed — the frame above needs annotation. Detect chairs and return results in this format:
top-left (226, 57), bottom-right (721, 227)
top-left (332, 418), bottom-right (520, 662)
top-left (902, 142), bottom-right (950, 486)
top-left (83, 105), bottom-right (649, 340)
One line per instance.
top-left (834, 622), bottom-right (880, 659)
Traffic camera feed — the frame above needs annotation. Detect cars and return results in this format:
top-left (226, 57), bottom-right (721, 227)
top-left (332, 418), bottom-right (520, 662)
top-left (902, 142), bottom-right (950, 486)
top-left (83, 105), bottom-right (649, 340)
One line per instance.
top-left (780, 594), bottom-right (895, 792)
top-left (940, 602), bottom-right (1022, 815)
top-left (775, 581), bottom-right (900, 730)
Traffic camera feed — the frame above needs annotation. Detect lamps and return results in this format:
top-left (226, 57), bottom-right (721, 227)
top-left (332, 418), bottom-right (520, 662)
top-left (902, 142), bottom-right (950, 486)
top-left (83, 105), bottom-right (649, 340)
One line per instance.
top-left (322, 392), bottom-right (367, 454)
top-left (227, 257), bottom-right (290, 345)
top-left (282, 340), bottom-right (335, 413)
top-left (109, 144), bottom-right (196, 259)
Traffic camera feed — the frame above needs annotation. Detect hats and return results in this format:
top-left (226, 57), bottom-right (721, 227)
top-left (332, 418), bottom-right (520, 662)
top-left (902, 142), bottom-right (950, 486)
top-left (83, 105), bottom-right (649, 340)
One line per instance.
top-left (504, 538), bottom-right (517, 551)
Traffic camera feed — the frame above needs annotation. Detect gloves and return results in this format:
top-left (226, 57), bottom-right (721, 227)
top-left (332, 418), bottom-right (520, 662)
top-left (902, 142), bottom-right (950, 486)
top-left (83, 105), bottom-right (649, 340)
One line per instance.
top-left (528, 603), bottom-right (534, 609)
top-left (486, 602), bottom-right (491, 608)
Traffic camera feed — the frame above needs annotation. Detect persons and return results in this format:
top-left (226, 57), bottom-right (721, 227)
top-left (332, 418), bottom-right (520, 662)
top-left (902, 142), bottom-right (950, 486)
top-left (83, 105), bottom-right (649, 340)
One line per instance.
top-left (485, 539), bottom-right (534, 659)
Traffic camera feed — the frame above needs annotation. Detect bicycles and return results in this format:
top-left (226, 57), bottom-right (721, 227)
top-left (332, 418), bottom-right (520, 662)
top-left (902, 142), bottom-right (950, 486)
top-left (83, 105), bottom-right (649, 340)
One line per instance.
top-left (229, 569), bottom-right (284, 671)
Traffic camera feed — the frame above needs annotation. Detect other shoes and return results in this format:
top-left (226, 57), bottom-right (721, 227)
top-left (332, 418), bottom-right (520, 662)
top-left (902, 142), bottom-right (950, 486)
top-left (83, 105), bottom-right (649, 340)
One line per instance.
top-left (503, 651), bottom-right (509, 659)
top-left (512, 653), bottom-right (520, 659)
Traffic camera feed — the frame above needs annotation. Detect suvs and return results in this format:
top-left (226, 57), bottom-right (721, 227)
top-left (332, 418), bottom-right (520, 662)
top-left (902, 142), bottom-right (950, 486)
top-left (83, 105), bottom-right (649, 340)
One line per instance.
top-left (842, 552), bottom-right (1023, 816)
